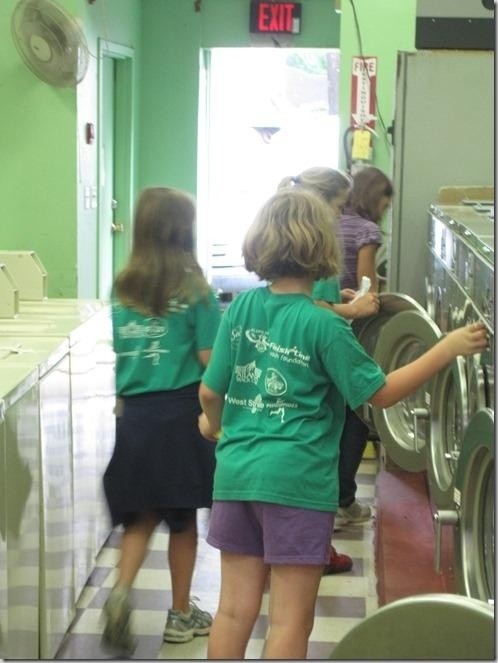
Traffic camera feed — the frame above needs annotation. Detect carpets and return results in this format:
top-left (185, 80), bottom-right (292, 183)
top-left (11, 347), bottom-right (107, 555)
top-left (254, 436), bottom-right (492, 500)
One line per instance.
top-left (373, 467), bottom-right (465, 609)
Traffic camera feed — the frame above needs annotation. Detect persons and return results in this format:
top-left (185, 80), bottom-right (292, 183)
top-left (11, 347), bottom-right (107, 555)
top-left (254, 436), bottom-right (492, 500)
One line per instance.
top-left (198, 188), bottom-right (489, 661)
top-left (98, 186), bottom-right (222, 654)
top-left (276, 166), bottom-right (352, 575)
top-left (332, 168), bottom-right (393, 530)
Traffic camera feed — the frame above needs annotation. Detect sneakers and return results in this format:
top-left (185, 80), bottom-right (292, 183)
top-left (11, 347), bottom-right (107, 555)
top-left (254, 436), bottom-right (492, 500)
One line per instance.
top-left (163, 604), bottom-right (212, 643)
top-left (100, 587), bottom-right (136, 657)
top-left (335, 501), bottom-right (372, 526)
top-left (325, 546), bottom-right (353, 573)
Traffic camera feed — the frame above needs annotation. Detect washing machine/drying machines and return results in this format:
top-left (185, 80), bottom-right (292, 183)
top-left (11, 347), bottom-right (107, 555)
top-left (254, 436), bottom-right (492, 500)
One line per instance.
top-left (331, 203), bottom-right (496, 660)
top-left (0, 249), bottom-right (117, 660)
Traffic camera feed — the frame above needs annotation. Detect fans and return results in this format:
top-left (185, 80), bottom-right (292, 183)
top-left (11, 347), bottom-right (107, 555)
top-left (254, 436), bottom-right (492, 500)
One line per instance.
top-left (9, 0), bottom-right (89, 90)
